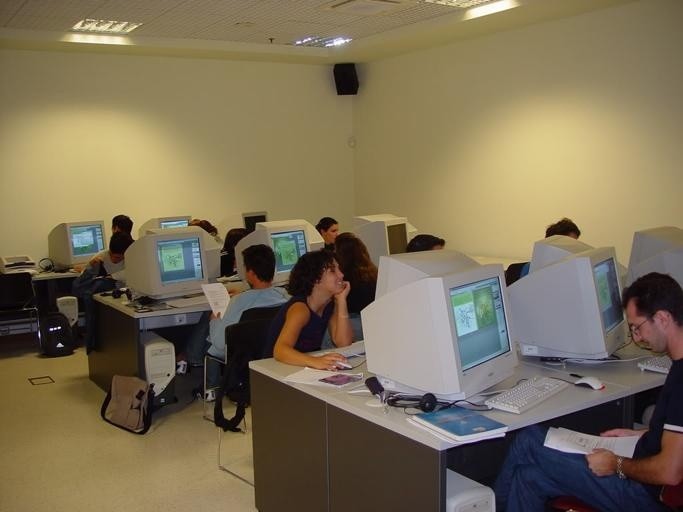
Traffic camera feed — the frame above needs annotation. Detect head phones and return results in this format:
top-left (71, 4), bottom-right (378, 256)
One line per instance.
top-left (112, 288), bottom-right (132, 299)
top-left (39, 258), bottom-right (56, 272)
top-left (386, 394), bottom-right (437, 412)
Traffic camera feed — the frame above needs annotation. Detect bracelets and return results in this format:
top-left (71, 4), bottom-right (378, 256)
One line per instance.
top-left (338, 313), bottom-right (349, 319)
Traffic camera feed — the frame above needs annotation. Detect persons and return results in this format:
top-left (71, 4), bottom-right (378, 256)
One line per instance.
top-left (404, 234), bottom-right (444, 251)
top-left (112, 215), bottom-right (133, 241)
top-left (220, 228), bottom-right (249, 277)
top-left (258, 247), bottom-right (353, 371)
top-left (189, 219), bottom-right (218, 238)
top-left (73, 231), bottom-right (134, 297)
top-left (315, 217), bottom-right (340, 251)
top-left (520, 219), bottom-right (580, 280)
top-left (492, 270), bottom-right (682, 511)
top-left (151, 244), bottom-right (292, 413)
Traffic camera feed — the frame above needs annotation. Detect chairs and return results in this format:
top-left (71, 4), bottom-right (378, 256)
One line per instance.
top-left (544, 479), bottom-right (683, 512)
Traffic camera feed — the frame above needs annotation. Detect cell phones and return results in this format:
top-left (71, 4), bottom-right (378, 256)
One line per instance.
top-left (160, 301), bottom-right (168, 310)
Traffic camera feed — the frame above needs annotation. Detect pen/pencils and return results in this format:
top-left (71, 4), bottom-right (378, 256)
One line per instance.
top-left (336, 361), bottom-right (352, 368)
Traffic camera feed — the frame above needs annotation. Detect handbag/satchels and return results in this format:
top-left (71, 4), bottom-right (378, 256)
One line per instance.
top-left (101, 375), bottom-right (154, 434)
top-left (225, 345), bottom-right (250, 403)
top-left (79, 273), bottom-right (116, 305)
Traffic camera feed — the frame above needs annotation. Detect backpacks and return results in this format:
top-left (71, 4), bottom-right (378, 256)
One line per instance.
top-left (40, 307), bottom-right (73, 356)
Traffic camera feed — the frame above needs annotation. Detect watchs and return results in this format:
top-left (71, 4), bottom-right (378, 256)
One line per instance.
top-left (614, 456), bottom-right (628, 480)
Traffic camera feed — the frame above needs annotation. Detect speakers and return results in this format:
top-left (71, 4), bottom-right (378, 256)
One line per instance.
top-left (333, 63), bottom-right (359, 95)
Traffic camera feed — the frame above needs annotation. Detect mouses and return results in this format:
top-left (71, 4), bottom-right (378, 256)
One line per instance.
top-left (574, 376), bottom-right (606, 391)
top-left (332, 360), bottom-right (353, 370)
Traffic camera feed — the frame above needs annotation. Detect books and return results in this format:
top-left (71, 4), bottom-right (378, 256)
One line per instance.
top-left (404, 406), bottom-right (510, 446)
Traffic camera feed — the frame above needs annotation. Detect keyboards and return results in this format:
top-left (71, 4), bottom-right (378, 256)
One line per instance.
top-left (484, 374), bottom-right (569, 415)
top-left (637, 352), bottom-right (673, 374)
top-left (313, 339), bottom-right (365, 358)
top-left (165, 295), bottom-right (209, 308)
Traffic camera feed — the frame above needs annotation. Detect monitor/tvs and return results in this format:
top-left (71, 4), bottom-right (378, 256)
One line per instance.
top-left (138, 215), bottom-right (192, 241)
top-left (506, 247), bottom-right (628, 365)
top-left (48, 220), bottom-right (109, 272)
top-left (125, 229), bottom-right (208, 300)
top-left (235, 224), bottom-right (311, 290)
top-left (625, 244), bottom-right (683, 292)
top-left (360, 263), bottom-right (520, 401)
top-left (375, 248), bottom-right (482, 301)
top-left (528, 235), bottom-right (628, 306)
top-left (350, 213), bottom-right (417, 246)
top-left (147, 226), bottom-right (221, 285)
top-left (242, 211), bottom-right (268, 233)
top-left (352, 217), bottom-right (409, 263)
top-left (629, 226), bottom-right (683, 267)
top-left (256, 218), bottom-right (325, 253)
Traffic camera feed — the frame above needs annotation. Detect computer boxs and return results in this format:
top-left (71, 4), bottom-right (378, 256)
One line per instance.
top-left (53, 292), bottom-right (81, 347)
top-left (446, 468), bottom-right (495, 512)
top-left (139, 331), bottom-right (176, 413)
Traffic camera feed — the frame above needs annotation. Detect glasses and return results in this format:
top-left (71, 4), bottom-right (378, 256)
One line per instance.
top-left (631, 317), bottom-right (649, 335)
